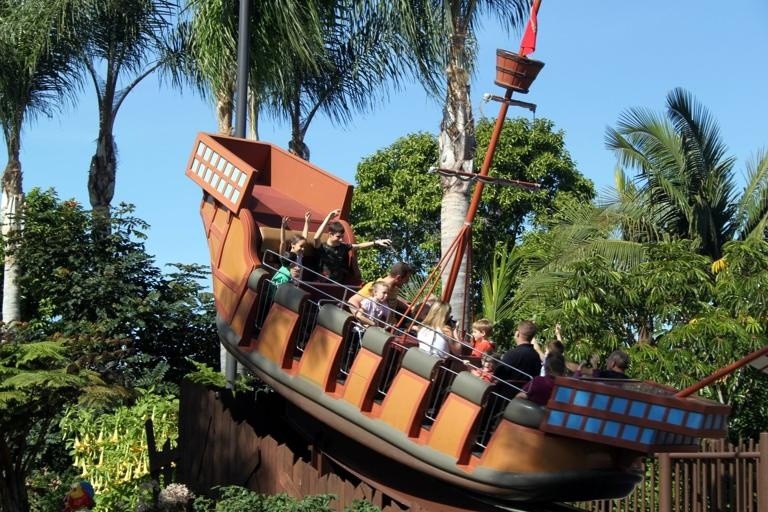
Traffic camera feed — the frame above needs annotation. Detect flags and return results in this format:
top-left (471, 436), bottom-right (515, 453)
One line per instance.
top-left (519, 0), bottom-right (540, 59)
